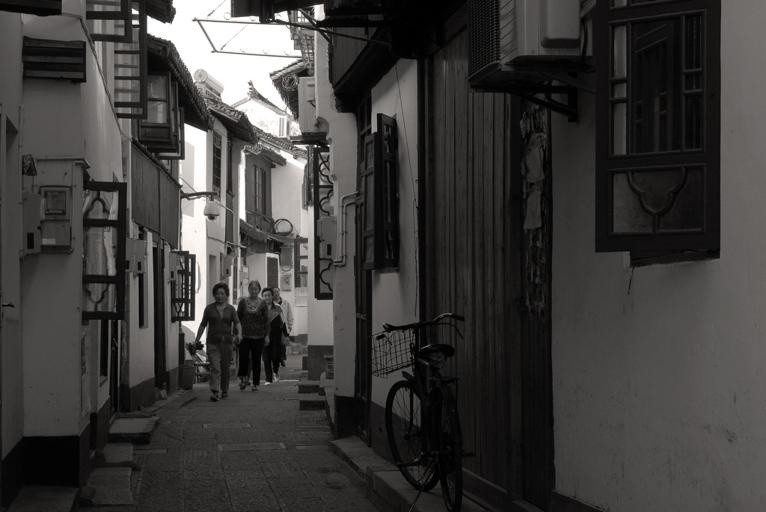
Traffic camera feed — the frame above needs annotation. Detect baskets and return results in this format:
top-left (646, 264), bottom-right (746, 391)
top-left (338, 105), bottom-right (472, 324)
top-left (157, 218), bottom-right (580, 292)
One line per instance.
top-left (369, 329), bottom-right (416, 379)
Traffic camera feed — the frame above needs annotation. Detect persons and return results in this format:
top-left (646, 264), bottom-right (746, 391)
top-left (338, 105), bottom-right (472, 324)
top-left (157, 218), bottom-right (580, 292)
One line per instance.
top-left (233, 280), bottom-right (268, 392)
top-left (192, 282), bottom-right (244, 401)
top-left (272, 287), bottom-right (294, 367)
top-left (260, 287), bottom-right (285, 386)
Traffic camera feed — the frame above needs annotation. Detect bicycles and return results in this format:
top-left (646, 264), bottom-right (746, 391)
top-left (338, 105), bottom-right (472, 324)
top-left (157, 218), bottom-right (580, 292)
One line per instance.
top-left (369, 313), bottom-right (466, 511)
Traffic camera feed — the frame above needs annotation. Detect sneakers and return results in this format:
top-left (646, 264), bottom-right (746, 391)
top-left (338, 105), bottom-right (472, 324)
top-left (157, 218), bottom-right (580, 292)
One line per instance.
top-left (239, 373), bottom-right (280, 392)
top-left (281, 360), bottom-right (285, 366)
top-left (210, 394), bottom-right (219, 402)
top-left (222, 391), bottom-right (227, 399)
